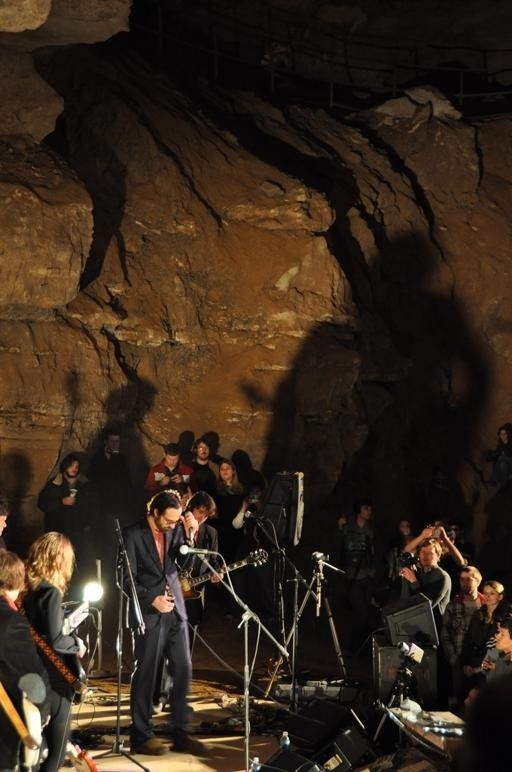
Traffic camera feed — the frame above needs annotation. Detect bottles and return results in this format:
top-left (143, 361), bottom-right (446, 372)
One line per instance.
top-left (279, 731), bottom-right (290, 748)
top-left (250, 756), bottom-right (260, 772)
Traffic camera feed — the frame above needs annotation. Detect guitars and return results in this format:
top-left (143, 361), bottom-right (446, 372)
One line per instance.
top-left (164, 548), bottom-right (270, 604)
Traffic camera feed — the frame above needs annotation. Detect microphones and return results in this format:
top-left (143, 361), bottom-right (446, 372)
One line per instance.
top-left (244, 510), bottom-right (270, 519)
top-left (179, 544), bottom-right (218, 555)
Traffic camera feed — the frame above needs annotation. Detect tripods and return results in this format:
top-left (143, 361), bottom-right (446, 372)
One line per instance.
top-left (263, 550), bottom-right (348, 699)
top-left (91, 518), bottom-right (152, 772)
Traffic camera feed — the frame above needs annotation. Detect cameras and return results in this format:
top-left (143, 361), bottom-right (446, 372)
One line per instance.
top-left (426, 524), bottom-right (441, 539)
top-left (107, 447), bottom-right (121, 456)
top-left (398, 552), bottom-right (419, 570)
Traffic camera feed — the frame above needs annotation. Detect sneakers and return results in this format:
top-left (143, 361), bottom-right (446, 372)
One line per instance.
top-left (131, 737), bottom-right (171, 756)
top-left (170, 733), bottom-right (205, 754)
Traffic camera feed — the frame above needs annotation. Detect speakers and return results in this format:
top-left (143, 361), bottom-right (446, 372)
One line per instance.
top-left (371, 630), bottom-right (439, 712)
top-left (381, 592), bottom-right (441, 647)
